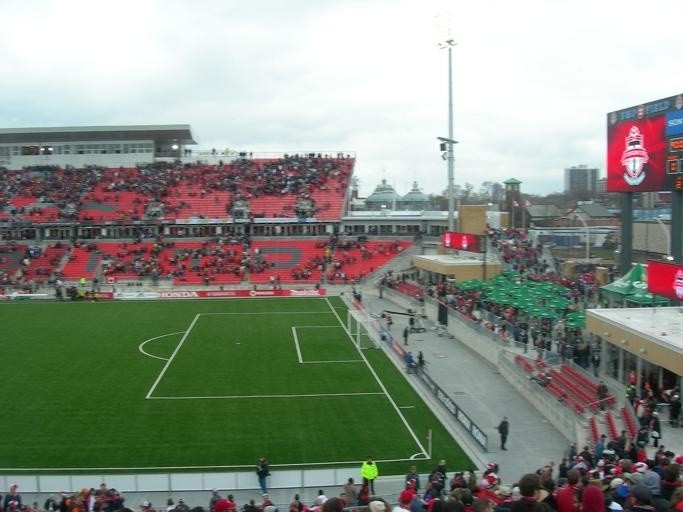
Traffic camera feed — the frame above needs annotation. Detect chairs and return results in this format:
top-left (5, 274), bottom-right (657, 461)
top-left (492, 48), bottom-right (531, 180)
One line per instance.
top-left (514, 349), bottom-right (645, 455)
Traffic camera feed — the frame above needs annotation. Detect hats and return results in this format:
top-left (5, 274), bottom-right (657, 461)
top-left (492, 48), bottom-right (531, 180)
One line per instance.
top-left (367, 500), bottom-right (387, 512)
top-left (8, 500), bottom-right (19, 507)
top-left (400, 430), bottom-right (683, 512)
top-left (9, 484), bottom-right (18, 488)
top-left (140, 488), bottom-right (270, 512)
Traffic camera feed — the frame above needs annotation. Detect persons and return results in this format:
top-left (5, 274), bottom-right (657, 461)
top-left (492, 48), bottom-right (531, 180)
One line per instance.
top-left (140, 490), bottom-right (347, 511)
top-left (349, 225), bottom-right (682, 511)
top-left (257, 458), bottom-right (268, 497)
top-left (0, 482), bottom-right (132, 512)
top-left (344, 478), bottom-right (357, 509)
top-left (1, 149), bottom-right (402, 304)
